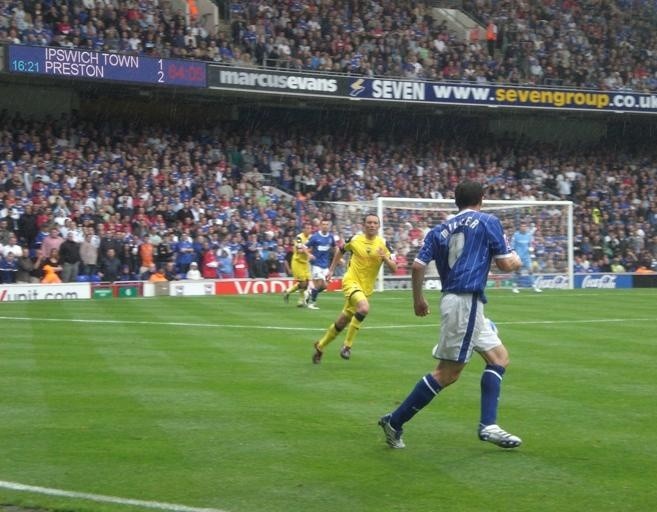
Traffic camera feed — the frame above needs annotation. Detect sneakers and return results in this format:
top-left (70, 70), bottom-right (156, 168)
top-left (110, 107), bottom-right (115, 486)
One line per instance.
top-left (282, 290), bottom-right (320, 310)
top-left (313, 341), bottom-right (323, 364)
top-left (378, 413), bottom-right (406, 448)
top-left (479, 422), bottom-right (522, 448)
top-left (339, 344), bottom-right (351, 359)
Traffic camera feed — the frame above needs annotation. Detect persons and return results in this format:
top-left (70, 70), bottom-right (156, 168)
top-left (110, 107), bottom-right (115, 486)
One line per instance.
top-left (377, 180), bottom-right (522, 448)
top-left (311, 213), bottom-right (396, 364)
top-left (0, 2), bottom-right (656, 284)
top-left (283, 222), bottom-right (316, 308)
top-left (303, 220), bottom-right (345, 309)
